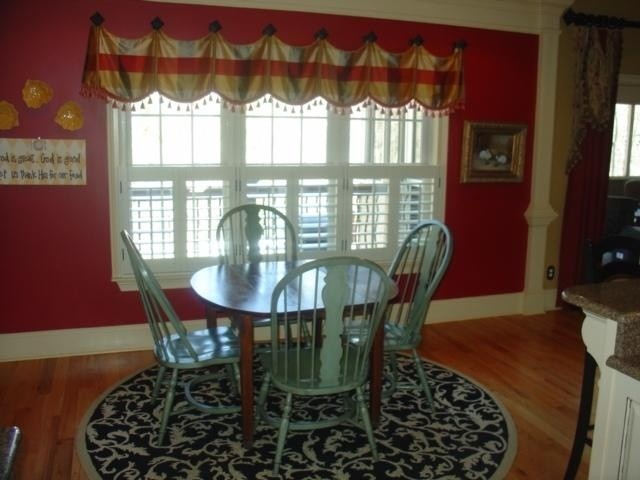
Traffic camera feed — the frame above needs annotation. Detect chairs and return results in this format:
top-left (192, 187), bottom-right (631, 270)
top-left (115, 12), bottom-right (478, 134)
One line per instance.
top-left (254, 255), bottom-right (391, 475)
top-left (565, 237), bottom-right (640, 480)
top-left (216, 201), bottom-right (312, 342)
top-left (120, 229), bottom-right (256, 447)
top-left (344, 219), bottom-right (454, 412)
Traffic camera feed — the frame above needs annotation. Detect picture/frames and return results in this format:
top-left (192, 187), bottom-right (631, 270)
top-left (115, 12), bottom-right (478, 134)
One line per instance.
top-left (458, 121), bottom-right (526, 188)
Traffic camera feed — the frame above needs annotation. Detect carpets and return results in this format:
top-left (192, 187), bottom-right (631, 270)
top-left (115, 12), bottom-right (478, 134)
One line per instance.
top-left (73, 332), bottom-right (521, 479)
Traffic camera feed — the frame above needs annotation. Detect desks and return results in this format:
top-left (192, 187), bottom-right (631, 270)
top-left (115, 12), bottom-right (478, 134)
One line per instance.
top-left (189, 259), bottom-right (399, 444)
